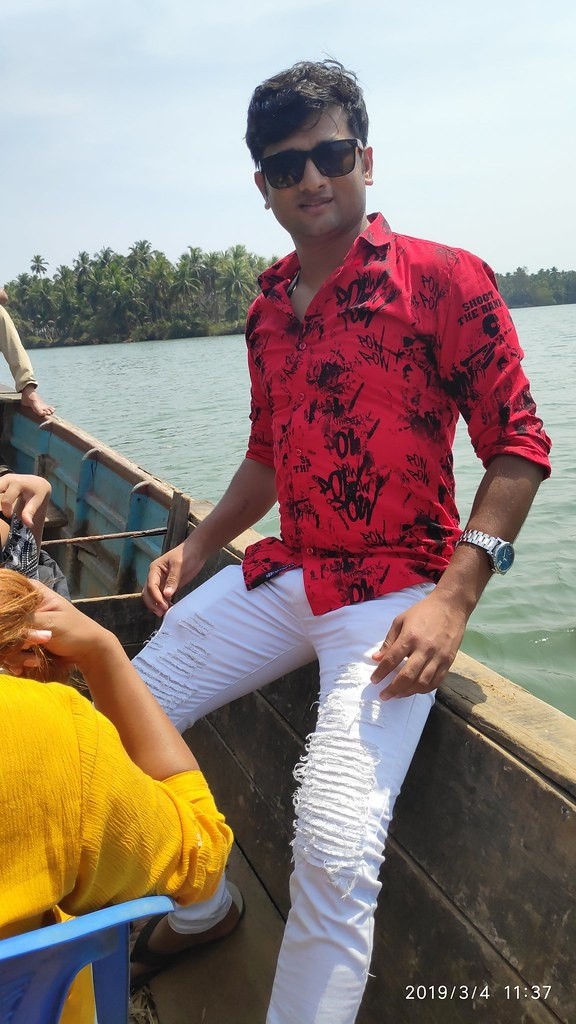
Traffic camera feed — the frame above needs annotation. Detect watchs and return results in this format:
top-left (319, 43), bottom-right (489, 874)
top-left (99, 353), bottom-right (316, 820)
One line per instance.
top-left (455, 531), bottom-right (516, 576)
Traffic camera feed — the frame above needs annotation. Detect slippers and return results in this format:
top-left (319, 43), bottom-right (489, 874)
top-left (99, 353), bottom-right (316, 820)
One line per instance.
top-left (129, 882), bottom-right (245, 995)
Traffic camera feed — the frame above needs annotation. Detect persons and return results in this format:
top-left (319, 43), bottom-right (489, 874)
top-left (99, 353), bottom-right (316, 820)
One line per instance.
top-left (0, 464), bottom-right (66, 595)
top-left (127, 60), bottom-right (551, 1023)
top-left (0, 291), bottom-right (53, 418)
top-left (0, 564), bottom-right (233, 1024)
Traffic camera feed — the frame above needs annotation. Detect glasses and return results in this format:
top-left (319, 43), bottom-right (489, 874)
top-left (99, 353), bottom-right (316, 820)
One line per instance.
top-left (259, 138), bottom-right (364, 190)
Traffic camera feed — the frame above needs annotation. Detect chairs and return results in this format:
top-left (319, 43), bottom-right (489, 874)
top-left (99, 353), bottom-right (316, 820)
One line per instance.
top-left (0, 893), bottom-right (176, 1024)
